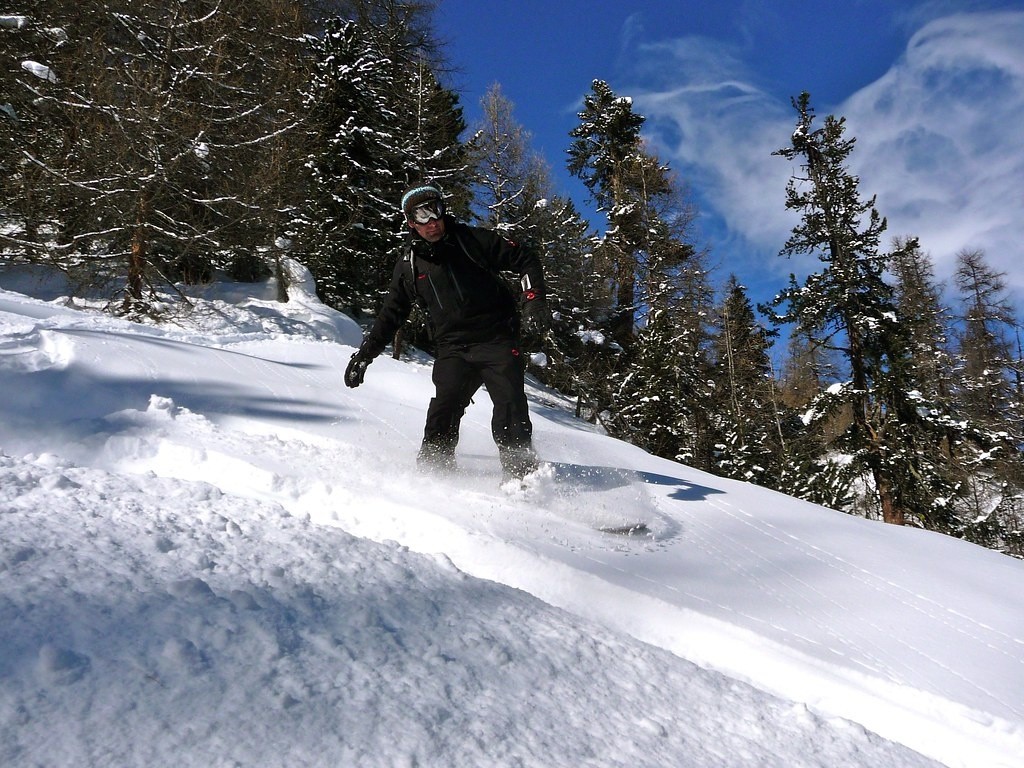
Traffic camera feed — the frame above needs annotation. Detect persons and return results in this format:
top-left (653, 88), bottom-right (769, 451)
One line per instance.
top-left (344, 187), bottom-right (545, 491)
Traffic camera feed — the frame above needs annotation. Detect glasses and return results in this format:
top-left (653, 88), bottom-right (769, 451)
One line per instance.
top-left (413, 199), bottom-right (448, 226)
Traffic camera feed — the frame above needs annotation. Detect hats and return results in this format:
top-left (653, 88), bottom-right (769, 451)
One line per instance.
top-left (402, 181), bottom-right (443, 222)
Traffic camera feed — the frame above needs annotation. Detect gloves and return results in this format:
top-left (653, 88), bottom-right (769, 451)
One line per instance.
top-left (344, 349), bottom-right (371, 390)
top-left (521, 291), bottom-right (549, 336)
